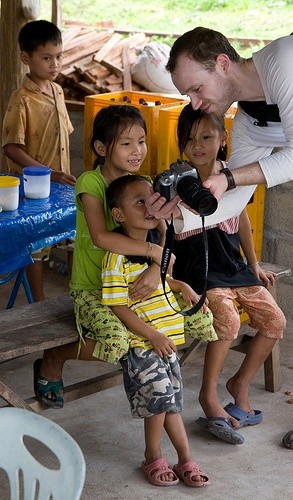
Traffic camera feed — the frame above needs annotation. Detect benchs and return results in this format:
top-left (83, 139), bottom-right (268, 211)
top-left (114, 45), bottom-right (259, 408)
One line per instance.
top-left (0, 253), bottom-right (292, 413)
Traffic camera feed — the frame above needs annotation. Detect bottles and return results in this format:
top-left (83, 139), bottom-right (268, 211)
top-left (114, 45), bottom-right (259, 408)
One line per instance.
top-left (49, 261), bottom-right (69, 274)
top-left (110, 96), bottom-right (161, 106)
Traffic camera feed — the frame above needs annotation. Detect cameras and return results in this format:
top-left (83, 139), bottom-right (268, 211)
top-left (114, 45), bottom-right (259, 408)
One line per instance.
top-left (152, 158), bottom-right (218, 216)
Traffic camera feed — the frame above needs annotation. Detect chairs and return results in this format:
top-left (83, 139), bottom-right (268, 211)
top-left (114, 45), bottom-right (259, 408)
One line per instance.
top-left (0, 404), bottom-right (86, 500)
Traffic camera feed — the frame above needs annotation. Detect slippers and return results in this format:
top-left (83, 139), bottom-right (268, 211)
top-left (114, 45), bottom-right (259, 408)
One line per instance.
top-left (196, 417), bottom-right (244, 445)
top-left (223, 402), bottom-right (263, 432)
top-left (33, 358), bottom-right (66, 409)
top-left (173, 461), bottom-right (209, 487)
top-left (141, 457), bottom-right (179, 486)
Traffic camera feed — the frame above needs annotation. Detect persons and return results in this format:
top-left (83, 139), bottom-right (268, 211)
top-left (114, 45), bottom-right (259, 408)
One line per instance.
top-left (33, 104), bottom-right (162, 409)
top-left (155, 102), bottom-right (287, 445)
top-left (144, 27), bottom-right (293, 449)
top-left (1, 20), bottom-right (77, 303)
top-left (99, 175), bottom-right (212, 488)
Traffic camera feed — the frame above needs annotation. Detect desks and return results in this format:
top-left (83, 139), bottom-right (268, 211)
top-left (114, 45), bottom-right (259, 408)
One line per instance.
top-left (0, 176), bottom-right (77, 311)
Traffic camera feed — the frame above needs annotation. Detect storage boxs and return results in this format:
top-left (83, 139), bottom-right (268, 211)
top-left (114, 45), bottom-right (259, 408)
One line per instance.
top-left (82, 90), bottom-right (191, 179)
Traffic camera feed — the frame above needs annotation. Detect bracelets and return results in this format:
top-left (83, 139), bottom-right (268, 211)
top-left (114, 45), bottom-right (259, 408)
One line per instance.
top-left (218, 167), bottom-right (236, 192)
top-left (248, 260), bottom-right (260, 267)
top-left (147, 240), bottom-right (152, 261)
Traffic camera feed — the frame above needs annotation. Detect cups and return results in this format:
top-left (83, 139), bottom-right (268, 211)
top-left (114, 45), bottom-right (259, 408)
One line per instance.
top-left (0, 176), bottom-right (20, 211)
top-left (22, 166), bottom-right (52, 200)
